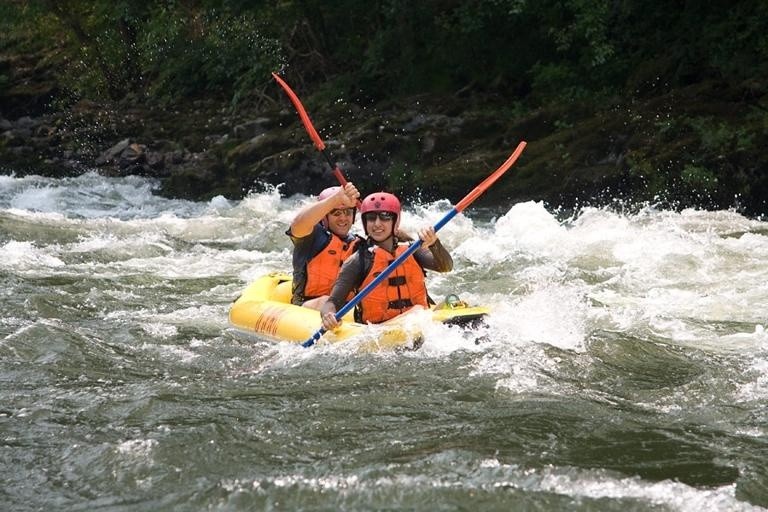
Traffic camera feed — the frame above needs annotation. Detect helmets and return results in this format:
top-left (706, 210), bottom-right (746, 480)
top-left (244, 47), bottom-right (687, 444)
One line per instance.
top-left (319, 186), bottom-right (357, 231)
top-left (361, 193), bottom-right (401, 237)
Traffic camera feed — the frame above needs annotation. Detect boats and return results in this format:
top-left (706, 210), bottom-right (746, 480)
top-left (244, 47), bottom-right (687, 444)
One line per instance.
top-left (230, 273), bottom-right (492, 347)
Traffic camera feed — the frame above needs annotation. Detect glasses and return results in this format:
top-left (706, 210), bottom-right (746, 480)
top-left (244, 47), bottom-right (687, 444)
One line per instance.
top-left (365, 211), bottom-right (394, 220)
top-left (330, 208), bottom-right (354, 217)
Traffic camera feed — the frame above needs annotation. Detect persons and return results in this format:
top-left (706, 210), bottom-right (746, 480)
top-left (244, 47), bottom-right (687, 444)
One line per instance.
top-left (285, 181), bottom-right (408, 312)
top-left (320, 192), bottom-right (453, 330)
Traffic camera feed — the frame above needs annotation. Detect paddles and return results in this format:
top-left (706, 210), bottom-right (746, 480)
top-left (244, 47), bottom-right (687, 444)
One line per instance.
top-left (273, 72), bottom-right (360, 208)
top-left (301, 142), bottom-right (527, 348)
